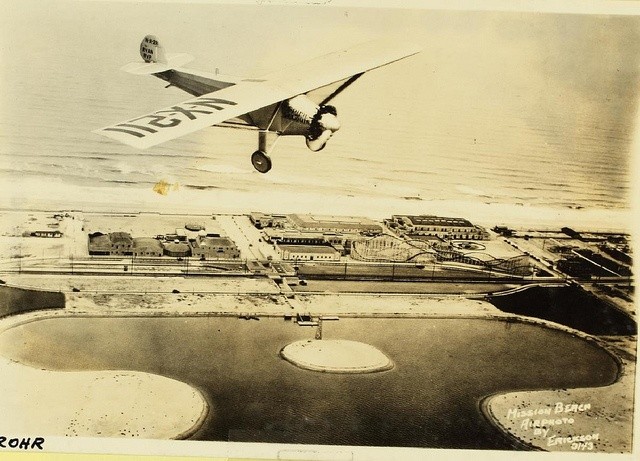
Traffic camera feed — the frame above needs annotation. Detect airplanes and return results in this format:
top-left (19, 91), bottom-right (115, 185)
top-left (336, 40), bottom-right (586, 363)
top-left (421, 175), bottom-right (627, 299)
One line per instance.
top-left (91, 34), bottom-right (422, 173)
